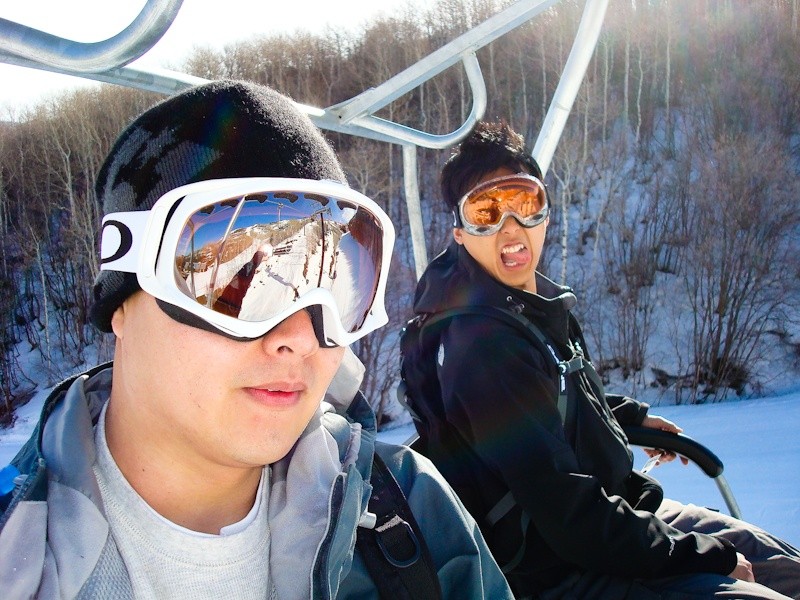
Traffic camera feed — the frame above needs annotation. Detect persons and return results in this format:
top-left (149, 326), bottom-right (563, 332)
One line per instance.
top-left (396, 115), bottom-right (800, 600)
top-left (0, 81), bottom-right (516, 600)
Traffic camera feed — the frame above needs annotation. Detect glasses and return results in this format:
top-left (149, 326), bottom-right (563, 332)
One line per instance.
top-left (452, 174), bottom-right (550, 236)
top-left (138, 177), bottom-right (395, 347)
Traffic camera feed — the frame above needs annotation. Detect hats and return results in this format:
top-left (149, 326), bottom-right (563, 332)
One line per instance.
top-left (91, 79), bottom-right (349, 334)
